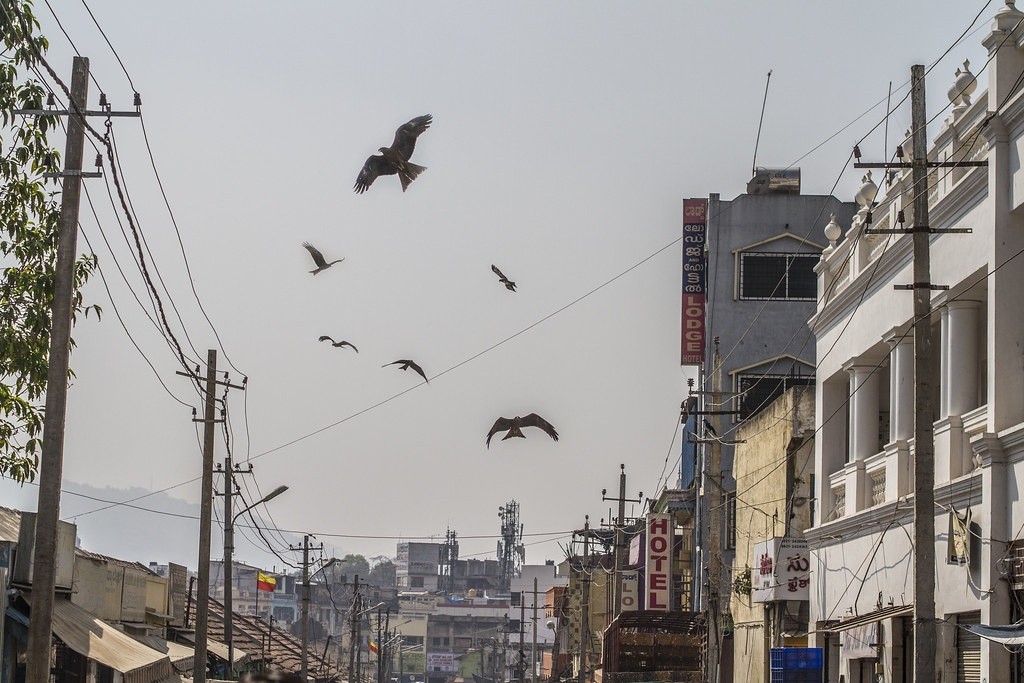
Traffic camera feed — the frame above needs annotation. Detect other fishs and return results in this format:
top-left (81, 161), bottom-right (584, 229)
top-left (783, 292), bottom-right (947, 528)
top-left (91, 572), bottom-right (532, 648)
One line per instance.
top-left (352, 114), bottom-right (435, 196)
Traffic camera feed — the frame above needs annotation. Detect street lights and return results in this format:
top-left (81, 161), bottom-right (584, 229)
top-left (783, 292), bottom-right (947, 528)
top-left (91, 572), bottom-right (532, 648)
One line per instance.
top-left (382, 618), bottom-right (422, 660)
top-left (349, 602), bottom-right (385, 683)
top-left (301, 557), bottom-right (336, 683)
top-left (219, 484), bottom-right (291, 641)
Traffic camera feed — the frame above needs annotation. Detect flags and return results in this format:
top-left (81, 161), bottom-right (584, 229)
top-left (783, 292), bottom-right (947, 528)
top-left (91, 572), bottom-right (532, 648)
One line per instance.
top-left (370, 641), bottom-right (378, 654)
top-left (258, 572), bottom-right (277, 592)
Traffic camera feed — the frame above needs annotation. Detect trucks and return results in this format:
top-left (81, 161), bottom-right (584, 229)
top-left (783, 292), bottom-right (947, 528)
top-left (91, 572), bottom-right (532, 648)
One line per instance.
top-left (590, 607), bottom-right (710, 683)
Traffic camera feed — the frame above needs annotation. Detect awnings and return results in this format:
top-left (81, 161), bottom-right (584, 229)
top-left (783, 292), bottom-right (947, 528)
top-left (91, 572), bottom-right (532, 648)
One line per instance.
top-left (179, 631), bottom-right (247, 669)
top-left (19, 593), bottom-right (195, 683)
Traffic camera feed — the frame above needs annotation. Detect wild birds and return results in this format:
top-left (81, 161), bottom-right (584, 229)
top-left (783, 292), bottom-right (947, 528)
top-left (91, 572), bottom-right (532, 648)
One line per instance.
top-left (490, 264), bottom-right (518, 293)
top-left (485, 413), bottom-right (560, 448)
top-left (380, 359), bottom-right (428, 384)
top-left (703, 418), bottom-right (717, 436)
top-left (318, 334), bottom-right (359, 353)
top-left (301, 241), bottom-right (344, 275)
